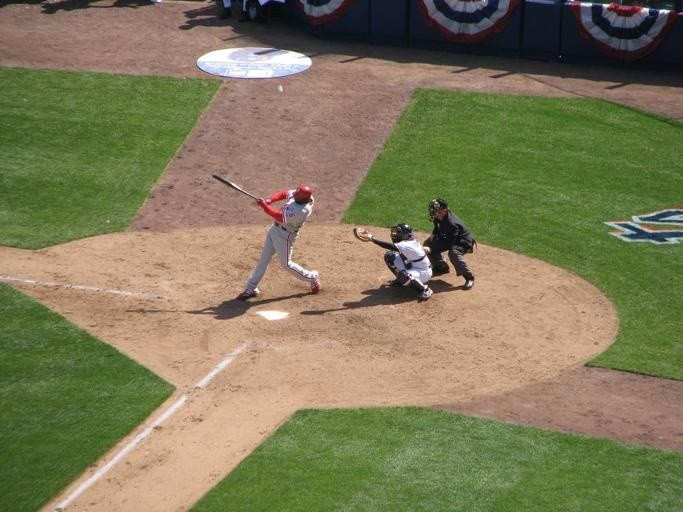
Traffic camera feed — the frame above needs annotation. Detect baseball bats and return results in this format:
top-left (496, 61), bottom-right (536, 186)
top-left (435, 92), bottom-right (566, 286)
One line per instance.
top-left (211, 174), bottom-right (257, 200)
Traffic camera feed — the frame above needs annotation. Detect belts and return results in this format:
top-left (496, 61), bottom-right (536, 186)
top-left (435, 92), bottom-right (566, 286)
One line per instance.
top-left (275, 222), bottom-right (286, 231)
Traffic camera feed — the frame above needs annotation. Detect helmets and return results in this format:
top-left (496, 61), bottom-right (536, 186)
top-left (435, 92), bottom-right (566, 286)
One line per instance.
top-left (429, 198), bottom-right (448, 221)
top-left (392, 224), bottom-right (411, 241)
top-left (292, 186), bottom-right (311, 201)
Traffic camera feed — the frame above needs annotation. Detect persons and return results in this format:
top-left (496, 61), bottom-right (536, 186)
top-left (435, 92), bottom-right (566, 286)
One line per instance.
top-left (216, 1), bottom-right (250, 24)
top-left (236, 183), bottom-right (320, 304)
top-left (422, 198), bottom-right (477, 291)
top-left (351, 221), bottom-right (434, 302)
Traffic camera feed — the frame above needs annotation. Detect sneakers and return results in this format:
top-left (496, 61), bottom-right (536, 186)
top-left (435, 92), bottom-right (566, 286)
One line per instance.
top-left (433, 267), bottom-right (449, 272)
top-left (463, 279), bottom-right (474, 288)
top-left (239, 289), bottom-right (258, 298)
top-left (419, 286), bottom-right (433, 299)
top-left (388, 279), bottom-right (400, 287)
top-left (310, 272), bottom-right (319, 293)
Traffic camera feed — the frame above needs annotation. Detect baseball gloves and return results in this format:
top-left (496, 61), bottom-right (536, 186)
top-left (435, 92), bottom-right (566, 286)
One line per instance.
top-left (353, 228), bottom-right (372, 241)
